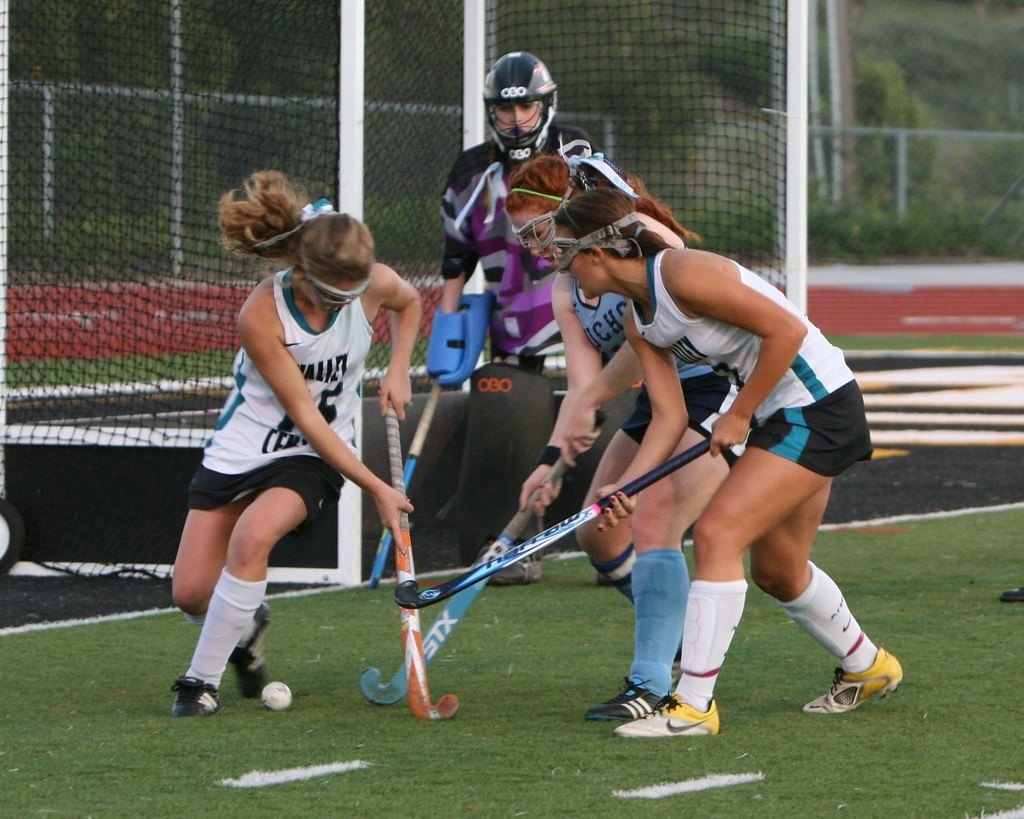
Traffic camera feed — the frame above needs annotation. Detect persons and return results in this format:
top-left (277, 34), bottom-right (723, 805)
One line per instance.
top-left (552, 189), bottom-right (903, 737)
top-left (504, 153), bottom-right (755, 721)
top-left (169, 168), bottom-right (424, 717)
top-left (423, 51), bottom-right (627, 585)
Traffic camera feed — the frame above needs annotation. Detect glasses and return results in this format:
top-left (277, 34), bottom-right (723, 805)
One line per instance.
top-left (552, 211), bottom-right (640, 271)
top-left (511, 154), bottom-right (573, 249)
top-left (308, 271), bottom-right (372, 305)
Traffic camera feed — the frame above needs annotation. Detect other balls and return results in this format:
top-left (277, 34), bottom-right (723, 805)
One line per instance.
top-left (260, 678), bottom-right (293, 712)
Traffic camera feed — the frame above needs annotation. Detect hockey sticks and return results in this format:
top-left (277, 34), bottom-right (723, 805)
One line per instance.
top-left (392, 438), bottom-right (713, 610)
top-left (385, 399), bottom-right (461, 720)
top-left (359, 407), bottom-right (607, 708)
top-left (366, 376), bottom-right (444, 590)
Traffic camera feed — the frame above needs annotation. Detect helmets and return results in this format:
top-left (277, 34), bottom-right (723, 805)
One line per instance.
top-left (482, 51), bottom-right (558, 162)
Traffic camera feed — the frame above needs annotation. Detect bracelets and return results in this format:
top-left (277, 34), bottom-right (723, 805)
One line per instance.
top-left (536, 446), bottom-right (561, 466)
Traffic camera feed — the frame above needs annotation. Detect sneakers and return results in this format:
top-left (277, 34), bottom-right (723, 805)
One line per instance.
top-left (584, 677), bottom-right (663, 721)
top-left (613, 693), bottom-right (721, 736)
top-left (803, 647), bottom-right (902, 714)
top-left (228, 602), bottom-right (272, 699)
top-left (170, 676), bottom-right (220, 717)
